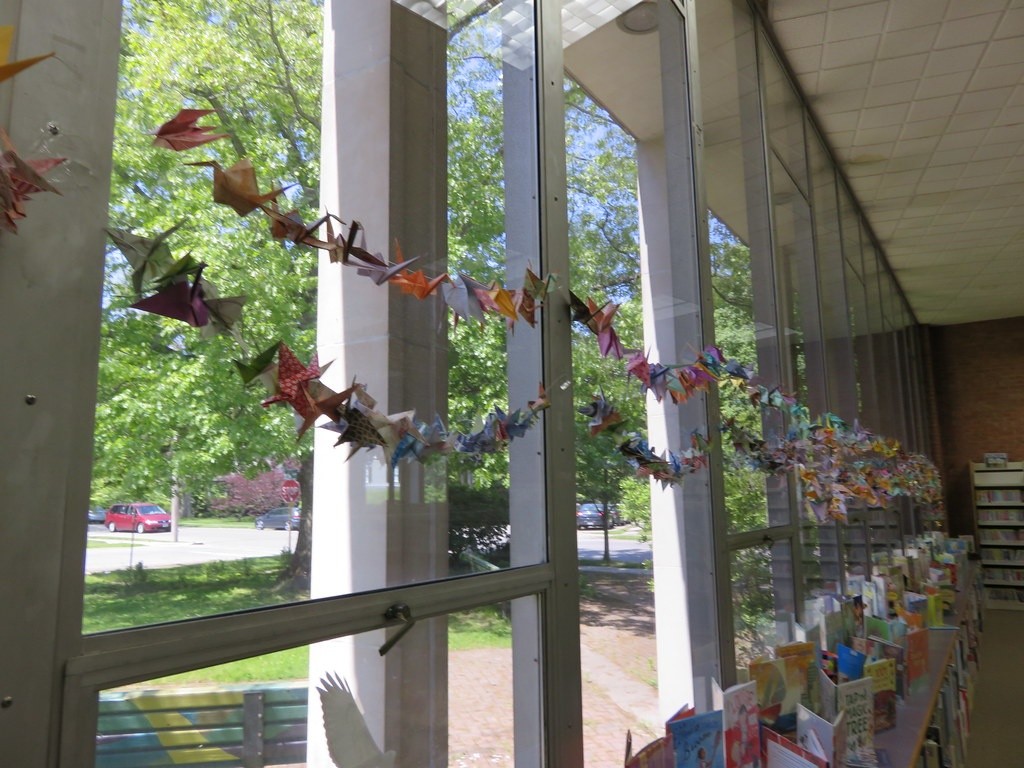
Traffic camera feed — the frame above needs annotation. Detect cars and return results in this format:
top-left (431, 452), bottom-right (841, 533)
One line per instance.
top-left (255, 504), bottom-right (302, 527)
top-left (88, 506), bottom-right (110, 524)
top-left (577, 504), bottom-right (627, 529)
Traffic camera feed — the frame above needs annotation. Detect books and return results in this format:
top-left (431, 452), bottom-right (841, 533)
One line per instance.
top-left (912, 576), bottom-right (984, 768)
top-left (978, 529), bottom-right (1024, 542)
top-left (977, 510), bottom-right (1024, 522)
top-left (625, 676), bottom-right (849, 768)
top-left (984, 568), bottom-right (1024, 582)
top-left (989, 589), bottom-right (1024, 603)
top-left (746, 609), bottom-right (897, 768)
top-left (975, 490), bottom-right (1021, 504)
top-left (833, 530), bottom-right (976, 701)
top-left (980, 549), bottom-right (1024, 561)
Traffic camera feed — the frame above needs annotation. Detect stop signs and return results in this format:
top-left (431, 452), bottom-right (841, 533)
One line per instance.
top-left (283, 479), bottom-right (299, 503)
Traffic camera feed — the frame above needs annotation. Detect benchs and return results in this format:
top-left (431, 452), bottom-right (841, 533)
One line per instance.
top-left (94, 678), bottom-right (308, 768)
top-left (468, 555), bottom-right (511, 618)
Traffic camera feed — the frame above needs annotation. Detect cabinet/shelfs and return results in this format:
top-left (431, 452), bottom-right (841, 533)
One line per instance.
top-left (874, 559), bottom-right (981, 768)
top-left (969, 460), bottom-right (1024, 611)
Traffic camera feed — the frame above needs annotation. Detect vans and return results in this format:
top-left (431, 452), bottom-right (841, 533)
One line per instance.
top-left (105, 503), bottom-right (171, 533)
